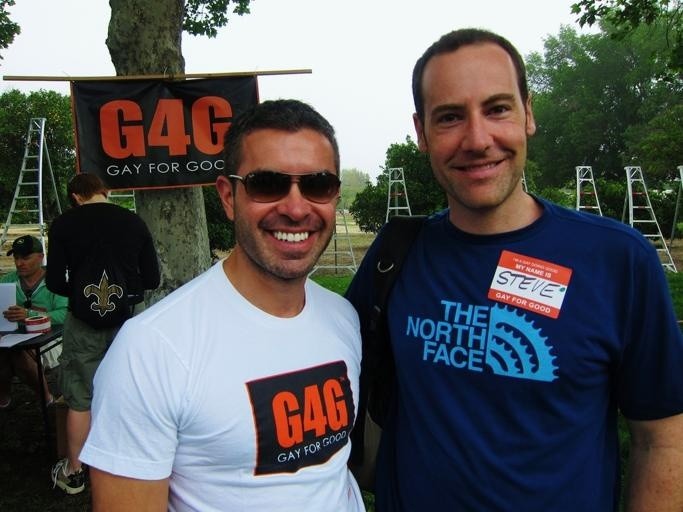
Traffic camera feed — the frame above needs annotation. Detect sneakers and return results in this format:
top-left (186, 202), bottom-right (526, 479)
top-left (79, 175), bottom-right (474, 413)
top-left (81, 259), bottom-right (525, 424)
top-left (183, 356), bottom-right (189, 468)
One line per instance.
top-left (48, 461), bottom-right (86, 495)
top-left (33, 391), bottom-right (56, 414)
top-left (1, 394), bottom-right (16, 411)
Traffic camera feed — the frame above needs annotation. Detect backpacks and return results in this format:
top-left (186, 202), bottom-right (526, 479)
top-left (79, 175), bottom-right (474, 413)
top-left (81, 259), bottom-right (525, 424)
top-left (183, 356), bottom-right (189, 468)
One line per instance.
top-left (60, 205), bottom-right (141, 327)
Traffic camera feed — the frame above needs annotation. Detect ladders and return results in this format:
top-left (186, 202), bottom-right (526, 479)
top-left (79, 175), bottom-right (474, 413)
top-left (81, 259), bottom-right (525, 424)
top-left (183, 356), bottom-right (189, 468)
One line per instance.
top-left (521, 170), bottom-right (529, 193)
top-left (1, 117), bottom-right (63, 268)
top-left (574, 166), bottom-right (603, 216)
top-left (386, 169), bottom-right (412, 222)
top-left (307, 186), bottom-right (356, 278)
top-left (622, 167), bottom-right (677, 275)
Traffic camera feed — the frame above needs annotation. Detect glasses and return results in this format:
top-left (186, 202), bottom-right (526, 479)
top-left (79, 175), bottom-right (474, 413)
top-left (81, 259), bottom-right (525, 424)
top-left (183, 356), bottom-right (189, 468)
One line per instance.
top-left (224, 171), bottom-right (340, 204)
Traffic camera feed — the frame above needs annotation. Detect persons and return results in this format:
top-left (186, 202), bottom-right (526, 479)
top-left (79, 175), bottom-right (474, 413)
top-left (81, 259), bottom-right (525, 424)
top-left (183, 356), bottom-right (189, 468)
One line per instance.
top-left (346, 28), bottom-right (683, 511)
top-left (45, 171), bottom-right (161, 495)
top-left (77, 99), bottom-right (368, 511)
top-left (0, 235), bottom-right (69, 407)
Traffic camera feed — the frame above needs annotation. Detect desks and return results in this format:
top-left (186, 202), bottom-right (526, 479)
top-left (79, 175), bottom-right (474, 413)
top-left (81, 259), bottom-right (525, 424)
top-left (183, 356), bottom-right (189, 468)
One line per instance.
top-left (0, 325), bottom-right (64, 451)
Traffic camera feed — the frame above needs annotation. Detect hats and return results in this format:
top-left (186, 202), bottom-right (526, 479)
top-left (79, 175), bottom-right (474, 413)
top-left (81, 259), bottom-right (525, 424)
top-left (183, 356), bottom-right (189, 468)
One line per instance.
top-left (5, 235), bottom-right (44, 257)
